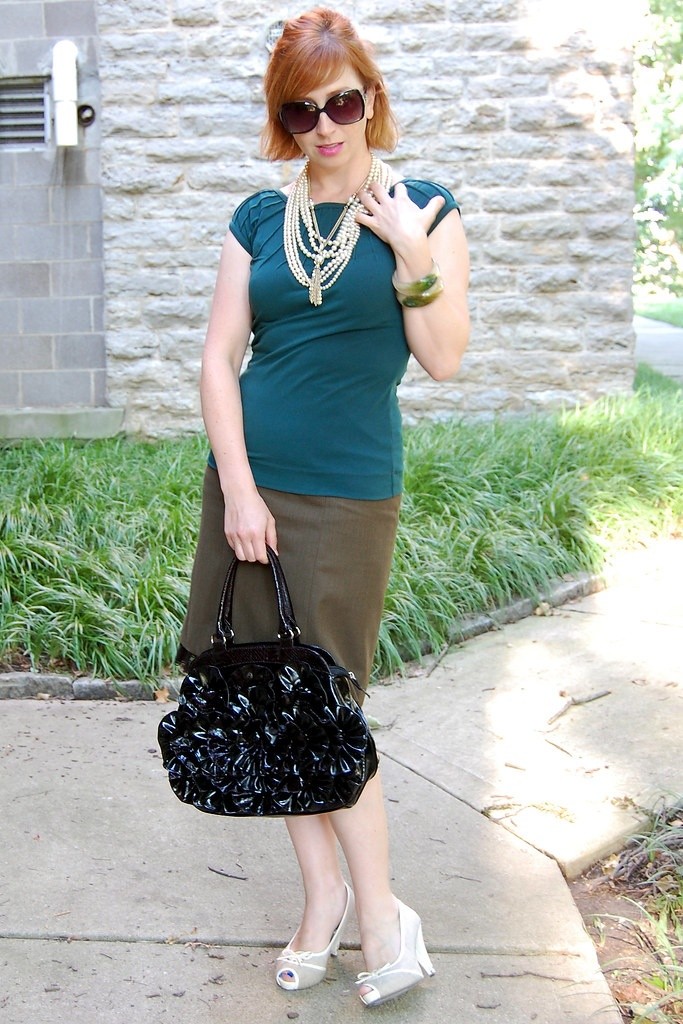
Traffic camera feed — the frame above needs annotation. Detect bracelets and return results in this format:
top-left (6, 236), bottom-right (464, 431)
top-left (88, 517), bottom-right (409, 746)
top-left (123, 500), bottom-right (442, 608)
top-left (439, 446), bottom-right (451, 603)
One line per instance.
top-left (391, 258), bottom-right (444, 308)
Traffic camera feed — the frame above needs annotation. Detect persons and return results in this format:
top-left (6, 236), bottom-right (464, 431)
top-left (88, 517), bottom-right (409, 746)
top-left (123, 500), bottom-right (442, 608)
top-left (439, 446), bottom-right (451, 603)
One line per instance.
top-left (178, 11), bottom-right (471, 1009)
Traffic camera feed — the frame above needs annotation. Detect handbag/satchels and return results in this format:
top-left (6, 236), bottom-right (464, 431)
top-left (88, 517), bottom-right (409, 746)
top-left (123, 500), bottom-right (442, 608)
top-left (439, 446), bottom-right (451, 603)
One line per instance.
top-left (158, 541), bottom-right (378, 816)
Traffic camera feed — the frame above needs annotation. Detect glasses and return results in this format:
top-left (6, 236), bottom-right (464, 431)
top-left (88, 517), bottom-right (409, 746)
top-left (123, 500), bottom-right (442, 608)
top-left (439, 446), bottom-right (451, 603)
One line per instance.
top-left (277, 85), bottom-right (368, 134)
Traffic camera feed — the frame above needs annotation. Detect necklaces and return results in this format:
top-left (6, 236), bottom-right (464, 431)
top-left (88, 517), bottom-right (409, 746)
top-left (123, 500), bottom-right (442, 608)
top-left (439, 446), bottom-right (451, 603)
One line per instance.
top-left (283, 154), bottom-right (395, 308)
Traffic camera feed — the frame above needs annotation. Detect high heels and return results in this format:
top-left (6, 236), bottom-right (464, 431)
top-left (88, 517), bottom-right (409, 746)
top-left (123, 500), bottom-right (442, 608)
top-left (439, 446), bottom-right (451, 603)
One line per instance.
top-left (273, 881), bottom-right (355, 990)
top-left (355, 894), bottom-right (436, 1006)
